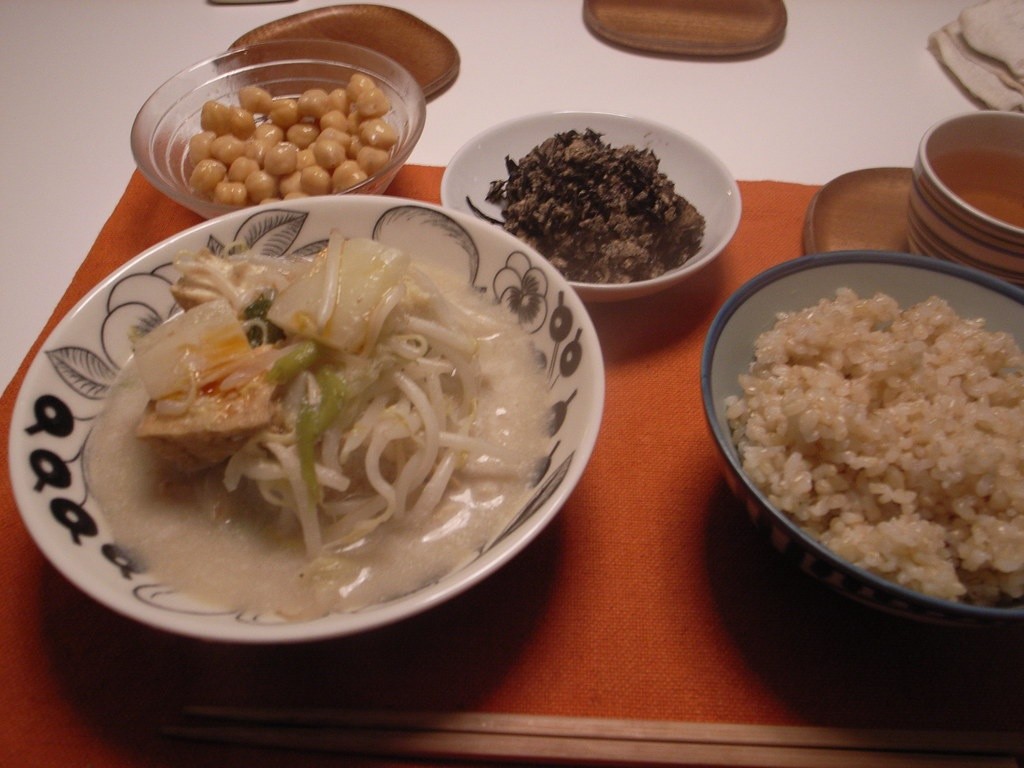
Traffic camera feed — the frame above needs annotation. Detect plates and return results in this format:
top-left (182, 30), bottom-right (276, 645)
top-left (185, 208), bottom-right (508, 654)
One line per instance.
top-left (583, 0), bottom-right (786, 56)
top-left (802, 168), bottom-right (913, 257)
top-left (226, 4), bottom-right (459, 104)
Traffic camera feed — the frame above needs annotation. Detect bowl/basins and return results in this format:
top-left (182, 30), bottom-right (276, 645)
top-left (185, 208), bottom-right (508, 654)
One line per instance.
top-left (7, 195), bottom-right (605, 650)
top-left (700, 248), bottom-right (1023, 632)
top-left (440, 111), bottom-right (743, 299)
top-left (130, 39), bottom-right (427, 223)
top-left (906, 110), bottom-right (1024, 293)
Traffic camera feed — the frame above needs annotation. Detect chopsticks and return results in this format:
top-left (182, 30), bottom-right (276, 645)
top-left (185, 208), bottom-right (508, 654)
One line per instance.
top-left (157, 703), bottom-right (1023, 768)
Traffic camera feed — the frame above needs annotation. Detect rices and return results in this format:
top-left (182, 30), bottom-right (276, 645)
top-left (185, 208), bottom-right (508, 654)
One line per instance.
top-left (722, 286), bottom-right (1024, 611)
